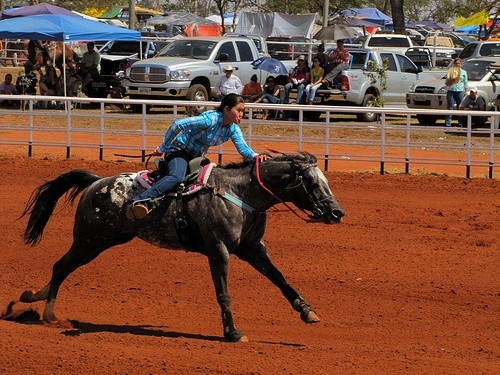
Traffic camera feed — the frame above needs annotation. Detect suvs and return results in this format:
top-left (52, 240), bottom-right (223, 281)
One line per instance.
top-left (85, 39), bottom-right (171, 109)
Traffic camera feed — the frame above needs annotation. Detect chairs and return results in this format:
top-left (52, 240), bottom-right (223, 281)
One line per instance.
top-left (263, 84), bottom-right (286, 119)
top-left (59, 73), bottom-right (82, 108)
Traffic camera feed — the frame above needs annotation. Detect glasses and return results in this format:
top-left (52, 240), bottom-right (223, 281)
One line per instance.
top-left (313, 60), bottom-right (319, 62)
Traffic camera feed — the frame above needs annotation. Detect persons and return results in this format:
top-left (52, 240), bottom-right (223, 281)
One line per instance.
top-left (496, 94), bottom-right (500, 135)
top-left (0, 39), bottom-right (101, 111)
top-left (444, 58), bottom-right (468, 127)
top-left (255, 76), bottom-right (280, 120)
top-left (214, 65), bottom-right (243, 102)
top-left (242, 74), bottom-right (263, 103)
top-left (283, 39), bottom-right (350, 105)
top-left (125, 93), bottom-right (258, 221)
top-left (457, 87), bottom-right (486, 127)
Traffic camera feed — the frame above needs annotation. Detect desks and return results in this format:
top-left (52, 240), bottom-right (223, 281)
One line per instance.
top-left (291, 88), bottom-right (343, 106)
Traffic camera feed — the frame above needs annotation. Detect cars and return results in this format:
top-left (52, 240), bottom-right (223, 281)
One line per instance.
top-left (222, 24), bottom-right (500, 59)
top-left (406, 57), bottom-right (500, 128)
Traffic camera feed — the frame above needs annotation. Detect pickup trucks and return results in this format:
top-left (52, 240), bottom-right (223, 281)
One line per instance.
top-left (120, 32), bottom-right (313, 117)
top-left (274, 47), bottom-right (421, 123)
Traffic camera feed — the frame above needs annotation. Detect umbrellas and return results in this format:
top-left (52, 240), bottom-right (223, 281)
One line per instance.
top-left (311, 24), bottom-right (360, 39)
top-left (251, 57), bottom-right (289, 74)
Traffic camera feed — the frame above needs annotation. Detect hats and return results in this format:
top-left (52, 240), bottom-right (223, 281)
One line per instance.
top-left (318, 44), bottom-right (325, 51)
top-left (447, 66), bottom-right (461, 84)
top-left (223, 65), bottom-right (235, 73)
top-left (297, 54), bottom-right (305, 62)
top-left (470, 86), bottom-right (478, 93)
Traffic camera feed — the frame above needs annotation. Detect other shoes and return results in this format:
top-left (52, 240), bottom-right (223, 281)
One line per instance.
top-left (124, 202), bottom-right (149, 221)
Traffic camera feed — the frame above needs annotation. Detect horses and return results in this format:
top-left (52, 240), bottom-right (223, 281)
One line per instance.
top-left (12, 147), bottom-right (344, 342)
top-left (36, 38), bottom-right (78, 71)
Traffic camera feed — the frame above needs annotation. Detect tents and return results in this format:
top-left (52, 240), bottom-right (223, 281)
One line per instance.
top-left (0, 3), bottom-right (83, 17)
top-left (0, 14), bottom-right (142, 112)
